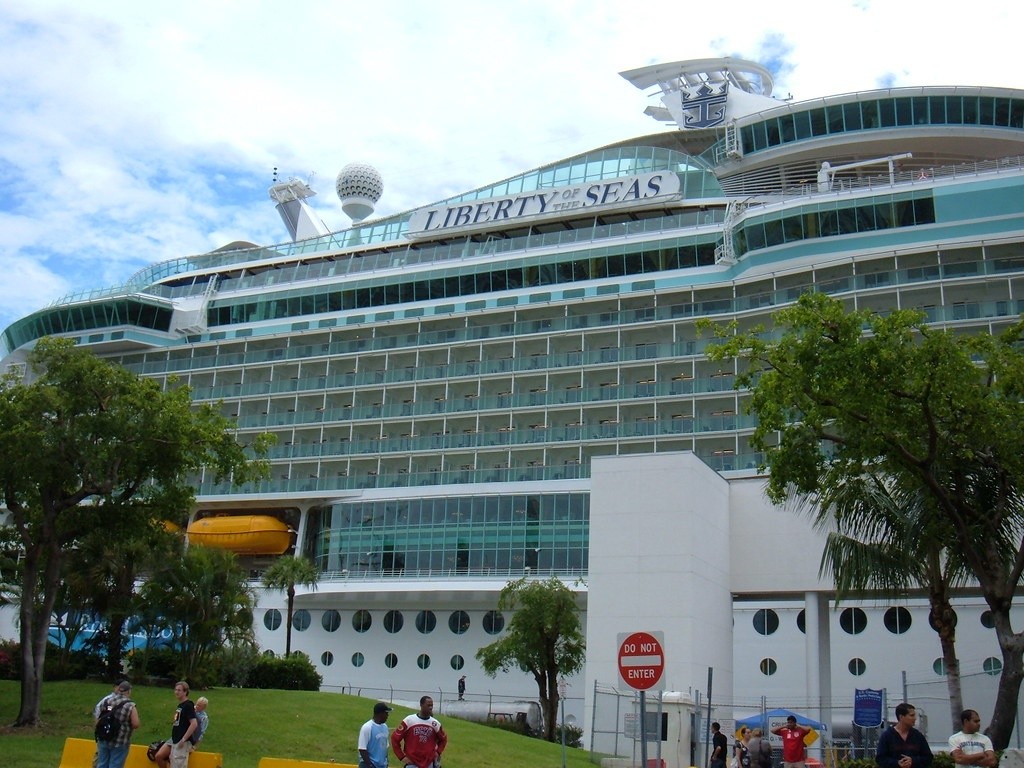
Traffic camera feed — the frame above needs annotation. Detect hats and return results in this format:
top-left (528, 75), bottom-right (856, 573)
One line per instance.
top-left (374, 703), bottom-right (392, 713)
top-left (115, 679), bottom-right (123, 685)
top-left (118, 681), bottom-right (133, 692)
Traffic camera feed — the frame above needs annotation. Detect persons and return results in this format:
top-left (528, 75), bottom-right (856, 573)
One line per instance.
top-left (155, 681), bottom-right (198, 768)
top-left (948, 709), bottom-right (996, 768)
top-left (93, 679), bottom-right (140, 768)
top-left (710, 722), bottom-right (727, 768)
top-left (736, 727), bottom-right (772, 768)
top-left (458, 675), bottom-right (466, 701)
top-left (194, 697), bottom-right (209, 741)
top-left (771, 715), bottom-right (810, 768)
top-left (875, 703), bottom-right (934, 768)
top-left (391, 696), bottom-right (447, 768)
top-left (842, 734), bottom-right (855, 760)
top-left (357, 702), bottom-right (392, 768)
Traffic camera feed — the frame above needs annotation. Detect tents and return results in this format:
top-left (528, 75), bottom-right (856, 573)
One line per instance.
top-left (735, 708), bottom-right (836, 768)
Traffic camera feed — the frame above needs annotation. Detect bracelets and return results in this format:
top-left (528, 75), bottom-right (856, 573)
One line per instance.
top-left (181, 738), bottom-right (185, 742)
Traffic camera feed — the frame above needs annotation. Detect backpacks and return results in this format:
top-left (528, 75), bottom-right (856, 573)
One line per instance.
top-left (95, 698), bottom-right (134, 741)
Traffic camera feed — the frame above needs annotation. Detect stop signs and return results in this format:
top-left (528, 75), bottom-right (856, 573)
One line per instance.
top-left (617, 633), bottom-right (665, 690)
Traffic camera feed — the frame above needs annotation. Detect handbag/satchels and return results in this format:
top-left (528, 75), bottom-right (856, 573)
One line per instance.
top-left (730, 742), bottom-right (742, 768)
top-left (759, 740), bottom-right (772, 768)
top-left (147, 740), bottom-right (165, 762)
